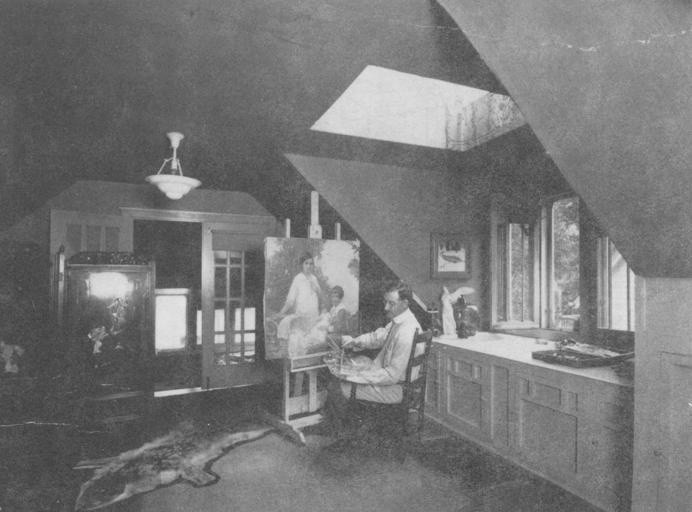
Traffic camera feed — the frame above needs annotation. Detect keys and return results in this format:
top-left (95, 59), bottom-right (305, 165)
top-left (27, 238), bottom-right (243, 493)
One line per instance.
top-left (329, 285), bottom-right (348, 330)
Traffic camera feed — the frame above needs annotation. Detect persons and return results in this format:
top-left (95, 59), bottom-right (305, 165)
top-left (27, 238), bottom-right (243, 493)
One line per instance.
top-left (304, 279), bottom-right (427, 452)
top-left (276, 250), bottom-right (321, 318)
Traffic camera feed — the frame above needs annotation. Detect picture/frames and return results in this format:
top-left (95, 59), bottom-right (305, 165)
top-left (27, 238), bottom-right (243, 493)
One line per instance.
top-left (429, 229), bottom-right (473, 281)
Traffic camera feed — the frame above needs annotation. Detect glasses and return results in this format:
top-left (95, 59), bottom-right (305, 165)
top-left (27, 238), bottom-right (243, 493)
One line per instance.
top-left (382, 300), bottom-right (406, 306)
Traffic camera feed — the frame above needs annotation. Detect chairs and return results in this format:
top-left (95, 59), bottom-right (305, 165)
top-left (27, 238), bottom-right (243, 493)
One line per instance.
top-left (331, 325), bottom-right (433, 440)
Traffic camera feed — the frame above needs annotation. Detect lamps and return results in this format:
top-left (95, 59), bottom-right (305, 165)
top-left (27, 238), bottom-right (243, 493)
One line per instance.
top-left (144, 131), bottom-right (203, 200)
